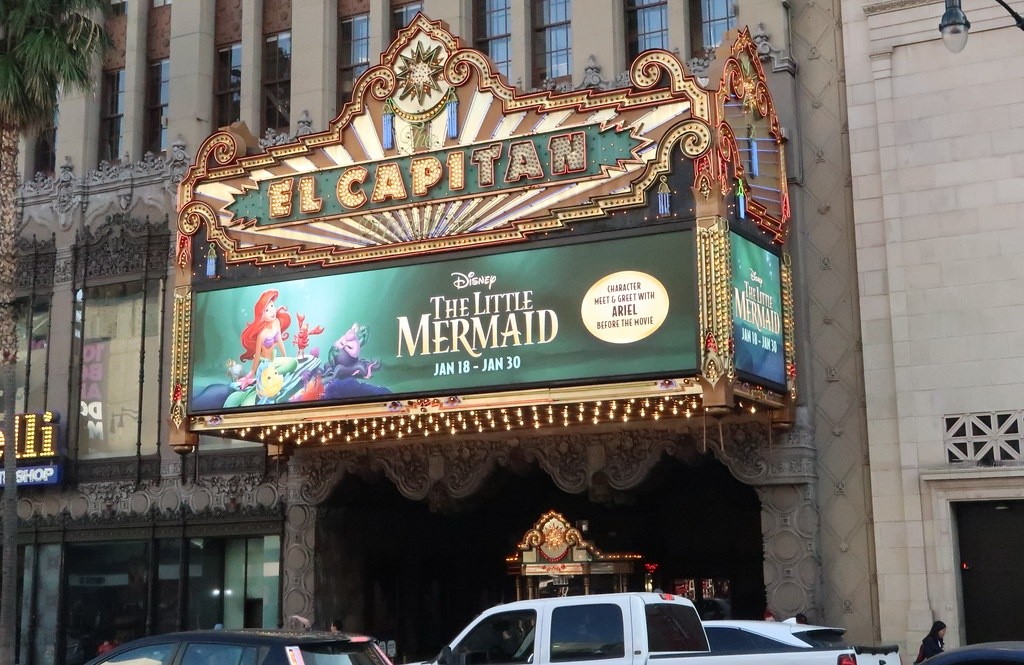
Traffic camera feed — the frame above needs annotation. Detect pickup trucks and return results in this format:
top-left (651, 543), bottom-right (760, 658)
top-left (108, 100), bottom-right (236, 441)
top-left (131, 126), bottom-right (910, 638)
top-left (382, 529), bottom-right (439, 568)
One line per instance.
top-left (404, 592), bottom-right (902, 665)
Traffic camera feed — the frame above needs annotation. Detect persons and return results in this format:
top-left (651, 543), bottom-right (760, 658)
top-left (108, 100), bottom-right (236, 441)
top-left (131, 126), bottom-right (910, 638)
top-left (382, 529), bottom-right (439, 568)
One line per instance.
top-left (96, 640), bottom-right (115, 656)
top-left (914, 621), bottom-right (947, 663)
top-left (501, 616), bottom-right (535, 662)
top-left (331, 620), bottom-right (343, 632)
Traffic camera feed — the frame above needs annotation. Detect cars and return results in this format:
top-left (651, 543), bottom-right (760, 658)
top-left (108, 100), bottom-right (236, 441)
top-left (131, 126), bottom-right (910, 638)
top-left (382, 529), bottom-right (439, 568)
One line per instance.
top-left (84, 628), bottom-right (391, 665)
top-left (65, 627), bottom-right (121, 665)
top-left (917, 641), bottom-right (1024, 665)
top-left (699, 617), bottom-right (846, 651)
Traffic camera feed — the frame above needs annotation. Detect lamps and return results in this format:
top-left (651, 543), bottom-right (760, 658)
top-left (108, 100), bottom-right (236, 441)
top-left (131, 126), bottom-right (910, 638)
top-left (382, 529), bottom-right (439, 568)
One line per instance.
top-left (938, 0), bottom-right (1024, 54)
